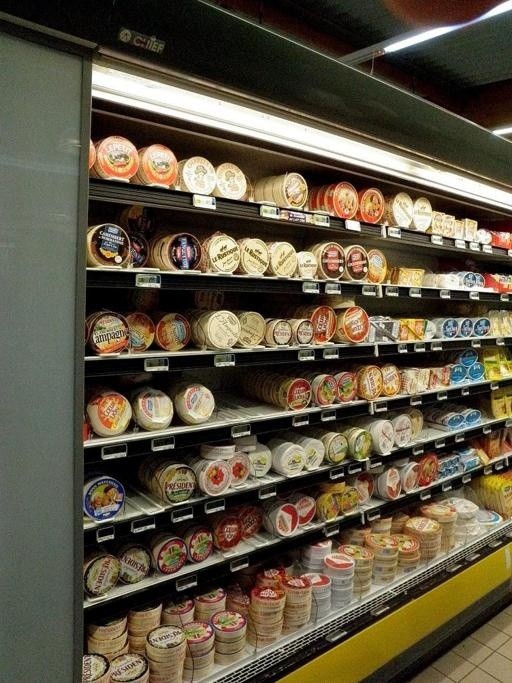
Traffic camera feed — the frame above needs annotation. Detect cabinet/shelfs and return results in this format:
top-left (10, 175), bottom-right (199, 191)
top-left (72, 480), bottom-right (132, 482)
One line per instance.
top-left (80, 98), bottom-right (512, 682)
